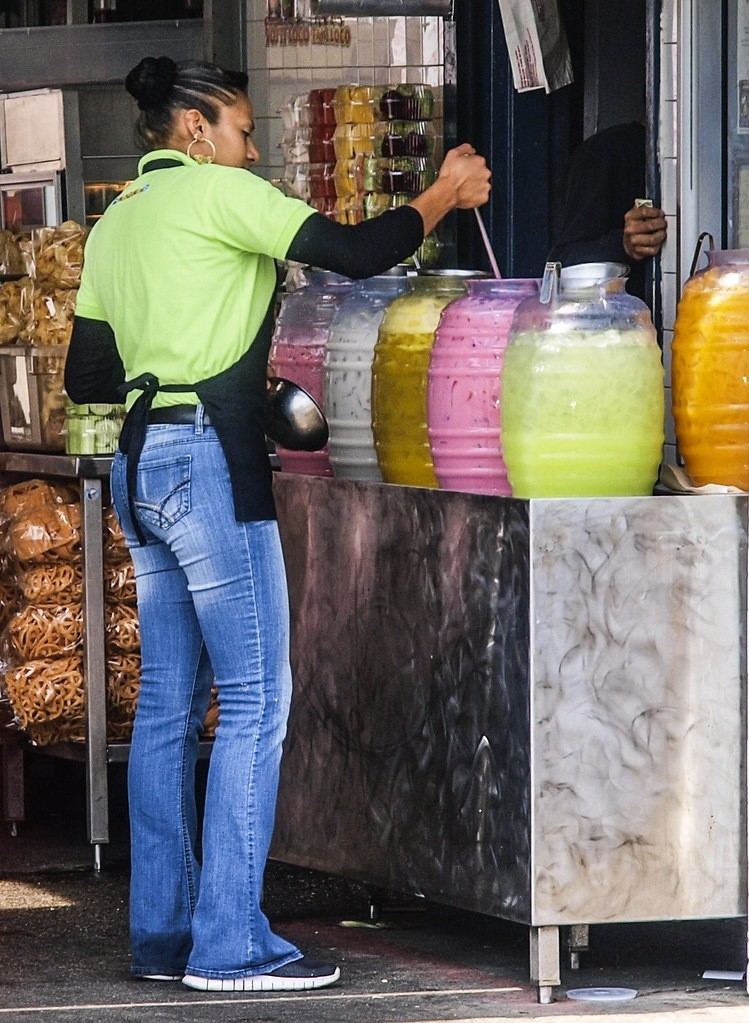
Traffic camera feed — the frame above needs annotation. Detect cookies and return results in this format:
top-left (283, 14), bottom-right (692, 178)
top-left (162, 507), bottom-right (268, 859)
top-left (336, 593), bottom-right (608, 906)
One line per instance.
top-left (0, 479), bottom-right (220, 745)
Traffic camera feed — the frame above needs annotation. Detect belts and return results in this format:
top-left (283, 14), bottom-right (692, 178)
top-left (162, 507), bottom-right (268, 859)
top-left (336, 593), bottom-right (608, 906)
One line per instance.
top-left (145, 403), bottom-right (211, 425)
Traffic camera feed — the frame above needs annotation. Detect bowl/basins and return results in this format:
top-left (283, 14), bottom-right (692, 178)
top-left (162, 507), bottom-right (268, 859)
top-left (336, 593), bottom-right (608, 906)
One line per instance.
top-left (264, 375), bottom-right (330, 452)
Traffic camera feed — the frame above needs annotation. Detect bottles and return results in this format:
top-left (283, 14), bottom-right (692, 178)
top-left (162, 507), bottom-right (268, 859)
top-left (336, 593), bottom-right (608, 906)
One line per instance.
top-left (671, 232), bottom-right (749, 495)
top-left (270, 259), bottom-right (665, 509)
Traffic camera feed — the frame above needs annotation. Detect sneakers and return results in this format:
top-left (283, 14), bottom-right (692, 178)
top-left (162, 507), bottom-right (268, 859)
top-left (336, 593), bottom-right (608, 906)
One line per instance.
top-left (140, 967), bottom-right (182, 981)
top-left (181, 954), bottom-right (340, 991)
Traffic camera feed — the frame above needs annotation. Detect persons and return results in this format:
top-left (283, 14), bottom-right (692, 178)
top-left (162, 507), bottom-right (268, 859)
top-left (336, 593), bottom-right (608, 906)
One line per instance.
top-left (536, 111), bottom-right (669, 277)
top-left (61, 56), bottom-right (492, 993)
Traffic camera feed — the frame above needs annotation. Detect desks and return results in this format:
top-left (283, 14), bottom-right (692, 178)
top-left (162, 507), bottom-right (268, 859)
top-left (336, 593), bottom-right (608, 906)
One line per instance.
top-left (0, 449), bottom-right (218, 878)
